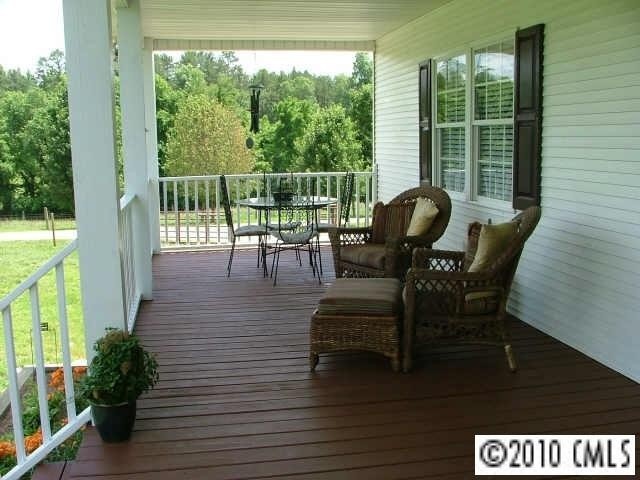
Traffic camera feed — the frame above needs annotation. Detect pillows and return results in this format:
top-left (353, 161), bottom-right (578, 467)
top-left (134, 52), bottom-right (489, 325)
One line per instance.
top-left (407, 197), bottom-right (438, 237)
top-left (465, 220), bottom-right (518, 301)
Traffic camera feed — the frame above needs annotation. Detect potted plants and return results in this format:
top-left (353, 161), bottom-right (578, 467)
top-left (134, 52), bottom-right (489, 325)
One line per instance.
top-left (80, 328), bottom-right (158, 445)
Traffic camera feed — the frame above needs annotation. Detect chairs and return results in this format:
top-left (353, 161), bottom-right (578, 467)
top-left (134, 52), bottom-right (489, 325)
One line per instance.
top-left (271, 178), bottom-right (323, 286)
top-left (400, 205), bottom-right (541, 372)
top-left (220, 173), bottom-right (270, 277)
top-left (328, 185), bottom-right (452, 281)
top-left (312, 170), bottom-right (354, 261)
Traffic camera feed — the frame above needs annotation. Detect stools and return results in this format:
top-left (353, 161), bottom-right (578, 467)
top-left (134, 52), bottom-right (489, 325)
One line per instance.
top-left (309, 278), bottom-right (402, 373)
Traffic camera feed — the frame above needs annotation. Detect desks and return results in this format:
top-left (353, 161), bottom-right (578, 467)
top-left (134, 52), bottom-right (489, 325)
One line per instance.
top-left (239, 196), bottom-right (337, 277)
